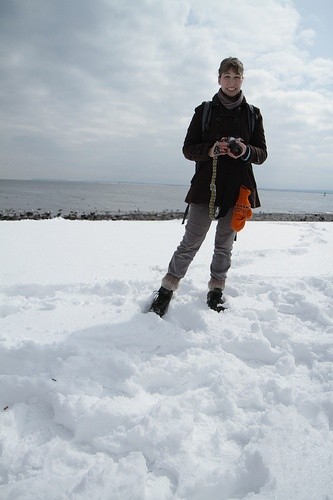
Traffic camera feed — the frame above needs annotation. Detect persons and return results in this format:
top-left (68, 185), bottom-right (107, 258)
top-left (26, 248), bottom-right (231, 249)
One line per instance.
top-left (150, 55), bottom-right (269, 315)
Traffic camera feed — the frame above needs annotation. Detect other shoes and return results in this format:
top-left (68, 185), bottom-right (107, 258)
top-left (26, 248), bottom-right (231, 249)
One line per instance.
top-left (149, 287), bottom-right (173, 318)
top-left (206, 288), bottom-right (227, 312)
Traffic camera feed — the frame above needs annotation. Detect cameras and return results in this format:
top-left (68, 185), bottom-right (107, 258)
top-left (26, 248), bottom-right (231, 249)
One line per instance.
top-left (227, 140), bottom-right (243, 157)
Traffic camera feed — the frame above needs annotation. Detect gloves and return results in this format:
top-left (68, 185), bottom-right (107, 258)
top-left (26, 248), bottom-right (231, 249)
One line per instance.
top-left (232, 184), bottom-right (251, 230)
